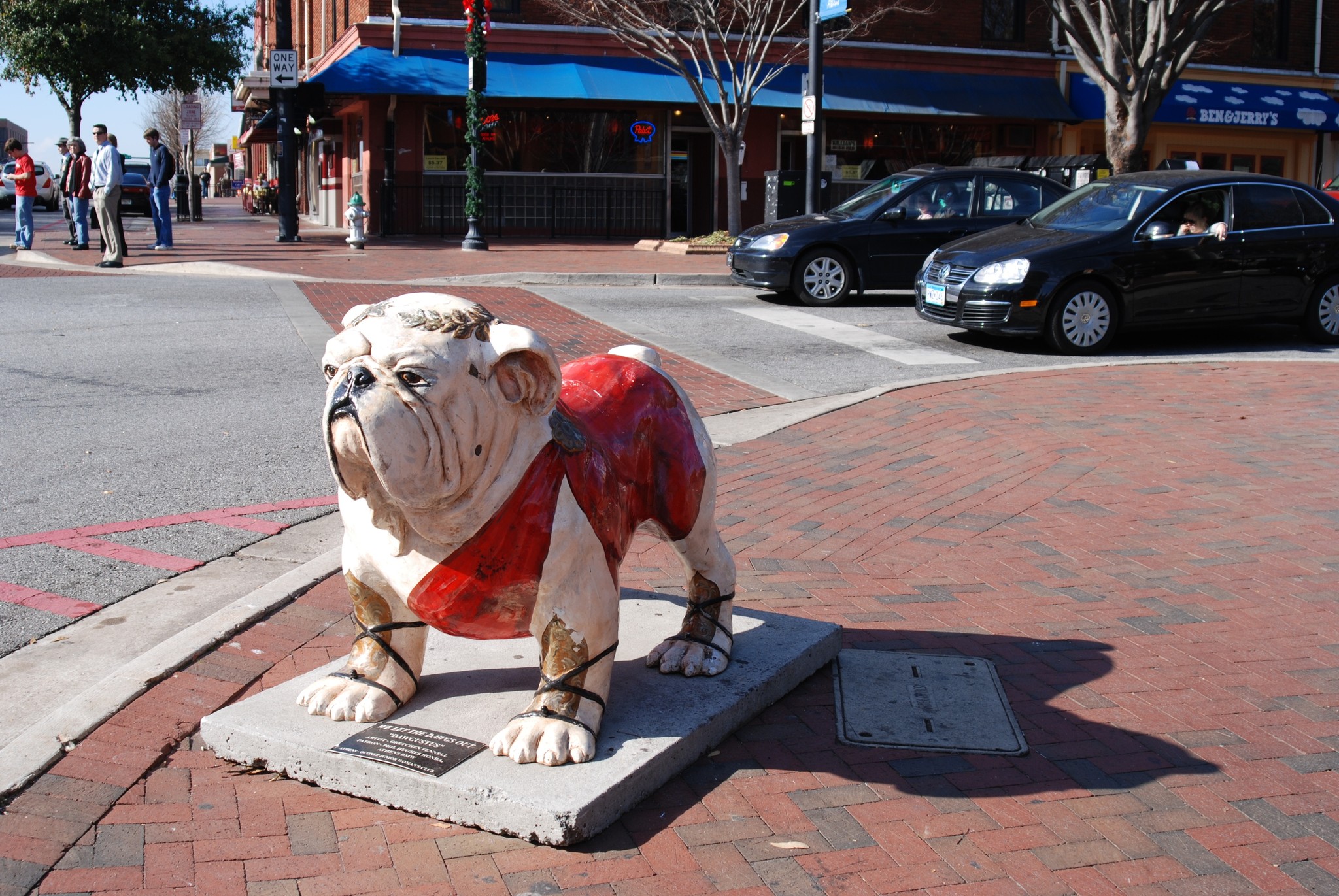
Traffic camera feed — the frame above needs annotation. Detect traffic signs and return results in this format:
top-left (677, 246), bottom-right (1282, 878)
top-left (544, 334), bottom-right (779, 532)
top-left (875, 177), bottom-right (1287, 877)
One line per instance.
top-left (269, 50), bottom-right (298, 87)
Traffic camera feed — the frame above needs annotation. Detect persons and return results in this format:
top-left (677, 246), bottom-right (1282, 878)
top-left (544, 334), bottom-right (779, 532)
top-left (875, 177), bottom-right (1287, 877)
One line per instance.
top-left (932, 182), bottom-right (968, 219)
top-left (1176, 200), bottom-right (1228, 242)
top-left (54, 138), bottom-right (78, 245)
top-left (913, 191), bottom-right (935, 219)
top-left (100, 133), bottom-right (129, 258)
top-left (4, 138), bottom-right (38, 250)
top-left (93, 124), bottom-right (124, 268)
top-left (252, 173), bottom-right (279, 216)
top-left (67, 136), bottom-right (92, 251)
top-left (143, 128), bottom-right (175, 250)
top-left (199, 167), bottom-right (211, 199)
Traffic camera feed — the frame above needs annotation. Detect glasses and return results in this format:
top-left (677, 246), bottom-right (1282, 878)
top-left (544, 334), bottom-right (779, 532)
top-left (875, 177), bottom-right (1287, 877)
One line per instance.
top-left (1186, 218), bottom-right (1201, 225)
top-left (93, 132), bottom-right (105, 135)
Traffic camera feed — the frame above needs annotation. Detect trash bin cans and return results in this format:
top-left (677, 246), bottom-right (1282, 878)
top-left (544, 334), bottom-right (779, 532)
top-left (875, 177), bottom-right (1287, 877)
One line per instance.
top-left (764, 167), bottom-right (833, 221)
top-left (968, 153), bottom-right (1030, 169)
top-left (1024, 155), bottom-right (1113, 189)
top-left (176, 174), bottom-right (203, 221)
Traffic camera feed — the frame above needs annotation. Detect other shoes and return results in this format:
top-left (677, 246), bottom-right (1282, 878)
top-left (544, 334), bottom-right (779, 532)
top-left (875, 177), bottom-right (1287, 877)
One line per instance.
top-left (16, 246), bottom-right (29, 250)
top-left (10, 244), bottom-right (18, 249)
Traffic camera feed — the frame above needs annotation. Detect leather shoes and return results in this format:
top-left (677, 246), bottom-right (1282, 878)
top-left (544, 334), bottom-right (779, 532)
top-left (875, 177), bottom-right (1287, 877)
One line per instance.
top-left (99, 261), bottom-right (123, 268)
top-left (94, 263), bottom-right (101, 266)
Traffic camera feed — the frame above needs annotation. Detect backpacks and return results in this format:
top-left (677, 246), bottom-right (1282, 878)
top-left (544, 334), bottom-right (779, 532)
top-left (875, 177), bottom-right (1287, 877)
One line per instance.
top-left (157, 146), bottom-right (175, 180)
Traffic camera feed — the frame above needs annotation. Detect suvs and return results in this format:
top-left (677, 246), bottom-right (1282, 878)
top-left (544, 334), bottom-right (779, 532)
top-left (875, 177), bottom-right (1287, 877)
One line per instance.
top-left (0, 160), bottom-right (60, 212)
top-left (124, 162), bottom-right (151, 181)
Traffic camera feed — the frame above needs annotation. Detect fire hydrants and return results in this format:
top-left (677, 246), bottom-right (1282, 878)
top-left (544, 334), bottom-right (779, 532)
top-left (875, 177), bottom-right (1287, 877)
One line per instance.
top-left (344, 191), bottom-right (369, 249)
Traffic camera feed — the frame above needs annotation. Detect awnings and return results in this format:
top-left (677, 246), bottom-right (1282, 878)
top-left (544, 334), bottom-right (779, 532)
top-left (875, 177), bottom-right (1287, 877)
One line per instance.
top-left (240, 122), bottom-right (275, 144)
top-left (254, 46), bottom-right (1078, 131)
top-left (1069, 70), bottom-right (1339, 132)
top-left (235, 130), bottom-right (249, 145)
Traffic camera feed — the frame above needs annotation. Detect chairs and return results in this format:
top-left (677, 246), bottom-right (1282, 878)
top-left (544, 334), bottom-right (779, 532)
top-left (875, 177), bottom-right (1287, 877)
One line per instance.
top-left (256, 186), bottom-right (278, 214)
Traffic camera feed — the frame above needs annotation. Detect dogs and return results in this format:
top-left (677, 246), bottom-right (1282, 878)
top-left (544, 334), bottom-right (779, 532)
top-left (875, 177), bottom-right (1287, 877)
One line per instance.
top-left (294, 292), bottom-right (735, 768)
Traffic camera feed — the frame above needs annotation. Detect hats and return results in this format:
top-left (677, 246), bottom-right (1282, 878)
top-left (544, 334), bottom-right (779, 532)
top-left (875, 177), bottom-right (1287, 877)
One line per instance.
top-left (55, 138), bottom-right (68, 145)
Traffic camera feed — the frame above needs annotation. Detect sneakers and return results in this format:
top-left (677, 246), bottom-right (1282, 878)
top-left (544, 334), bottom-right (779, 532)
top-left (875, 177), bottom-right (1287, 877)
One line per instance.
top-left (72, 244), bottom-right (88, 250)
top-left (148, 244), bottom-right (156, 249)
top-left (68, 241), bottom-right (78, 245)
top-left (63, 240), bottom-right (71, 244)
top-left (155, 244), bottom-right (172, 250)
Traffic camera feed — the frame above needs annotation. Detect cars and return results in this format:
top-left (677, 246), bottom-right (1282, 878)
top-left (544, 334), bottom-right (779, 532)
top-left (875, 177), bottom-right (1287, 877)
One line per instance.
top-left (913, 171), bottom-right (1339, 355)
top-left (1321, 174), bottom-right (1339, 201)
top-left (120, 173), bottom-right (152, 217)
top-left (726, 168), bottom-right (1125, 307)
